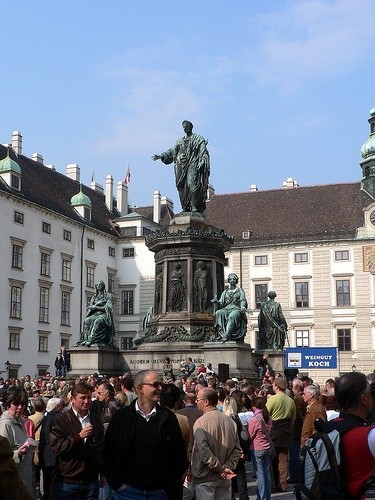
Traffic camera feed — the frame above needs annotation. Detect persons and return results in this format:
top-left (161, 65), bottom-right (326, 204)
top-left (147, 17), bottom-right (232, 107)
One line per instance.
top-left (76, 280), bottom-right (114, 345)
top-left (154, 261), bottom-right (210, 316)
top-left (259, 291), bottom-right (287, 349)
top-left (163, 357), bottom-right (173, 377)
top-left (186, 358), bottom-right (195, 377)
top-left (199, 364), bottom-right (206, 373)
top-left (0, 369), bottom-right (375, 500)
top-left (255, 352), bottom-right (269, 379)
top-left (206, 363), bottom-right (214, 373)
top-left (212, 273), bottom-right (247, 341)
top-left (151, 120), bottom-right (210, 212)
top-left (142, 307), bottom-right (153, 330)
top-left (54, 346), bottom-right (69, 378)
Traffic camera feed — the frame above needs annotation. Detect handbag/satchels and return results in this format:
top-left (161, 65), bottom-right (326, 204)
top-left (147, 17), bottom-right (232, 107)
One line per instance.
top-left (255, 359), bottom-right (262, 368)
top-left (269, 447), bottom-right (277, 461)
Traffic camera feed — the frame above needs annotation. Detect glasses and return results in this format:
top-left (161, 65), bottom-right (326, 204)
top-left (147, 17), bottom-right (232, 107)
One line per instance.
top-left (98, 391), bottom-right (104, 395)
top-left (366, 387), bottom-right (374, 395)
top-left (196, 398), bottom-right (204, 402)
top-left (12, 401), bottom-right (27, 407)
top-left (141, 381), bottom-right (163, 388)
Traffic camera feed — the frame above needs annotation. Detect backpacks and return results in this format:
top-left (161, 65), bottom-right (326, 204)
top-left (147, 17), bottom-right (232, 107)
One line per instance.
top-left (294, 417), bottom-right (375, 500)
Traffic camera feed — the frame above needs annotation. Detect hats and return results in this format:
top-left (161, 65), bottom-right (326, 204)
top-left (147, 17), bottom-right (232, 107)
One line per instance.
top-left (275, 377), bottom-right (287, 390)
top-left (334, 372), bottom-right (367, 411)
top-left (46, 398), bottom-right (61, 412)
top-left (232, 378), bottom-right (239, 384)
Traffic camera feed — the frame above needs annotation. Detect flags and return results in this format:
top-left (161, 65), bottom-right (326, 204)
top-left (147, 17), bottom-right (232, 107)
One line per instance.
top-left (124, 166), bottom-right (131, 184)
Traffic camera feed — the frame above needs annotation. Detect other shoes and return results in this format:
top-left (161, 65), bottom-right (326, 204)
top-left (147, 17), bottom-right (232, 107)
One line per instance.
top-left (271, 488), bottom-right (277, 493)
top-left (252, 475), bottom-right (256, 478)
